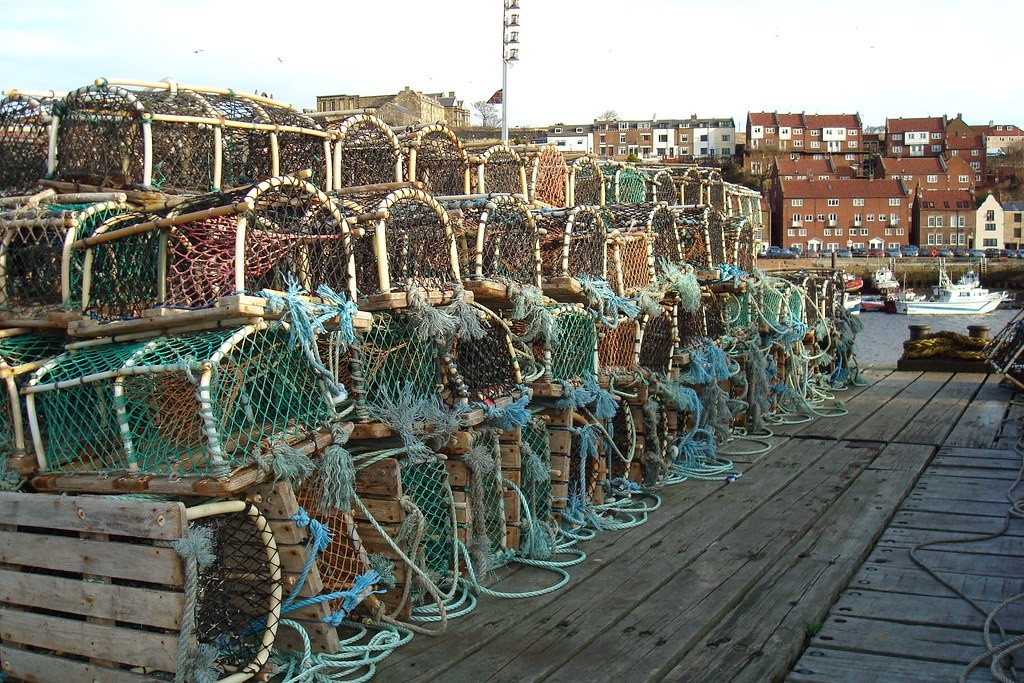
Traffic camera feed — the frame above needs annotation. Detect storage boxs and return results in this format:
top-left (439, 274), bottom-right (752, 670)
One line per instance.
top-left (0, 77), bottom-right (856, 683)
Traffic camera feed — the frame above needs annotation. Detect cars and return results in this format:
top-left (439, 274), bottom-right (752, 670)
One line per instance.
top-left (757, 246), bottom-right (1024, 260)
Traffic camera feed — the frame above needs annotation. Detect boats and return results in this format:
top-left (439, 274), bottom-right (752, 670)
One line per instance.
top-left (892, 255), bottom-right (1010, 315)
top-left (829, 269), bottom-right (927, 316)
top-left (871, 266), bottom-right (899, 292)
